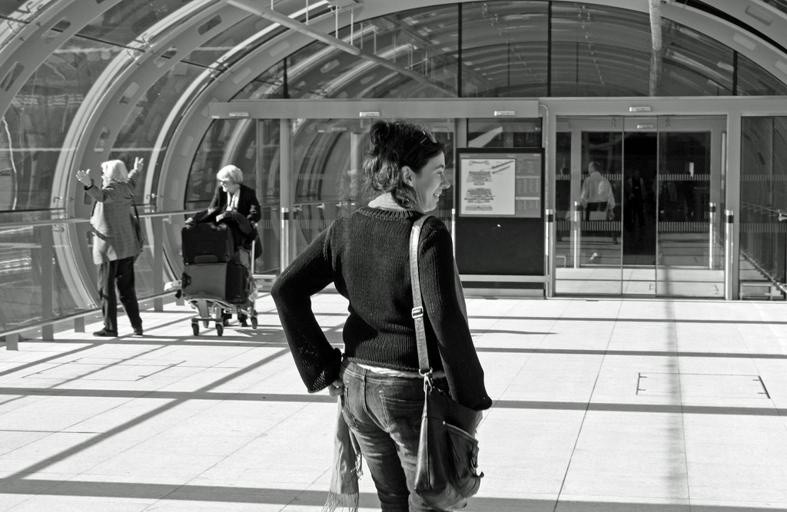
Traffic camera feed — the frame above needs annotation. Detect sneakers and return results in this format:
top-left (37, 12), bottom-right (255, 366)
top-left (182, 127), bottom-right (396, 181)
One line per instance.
top-left (93, 330), bottom-right (118, 337)
top-left (133, 328), bottom-right (143, 335)
top-left (589, 253), bottom-right (599, 263)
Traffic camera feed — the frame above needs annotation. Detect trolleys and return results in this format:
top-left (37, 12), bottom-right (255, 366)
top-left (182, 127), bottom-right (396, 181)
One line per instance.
top-left (175, 221), bottom-right (259, 336)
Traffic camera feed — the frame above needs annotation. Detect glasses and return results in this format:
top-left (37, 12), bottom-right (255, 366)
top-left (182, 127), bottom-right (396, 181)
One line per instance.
top-left (404, 127), bottom-right (437, 162)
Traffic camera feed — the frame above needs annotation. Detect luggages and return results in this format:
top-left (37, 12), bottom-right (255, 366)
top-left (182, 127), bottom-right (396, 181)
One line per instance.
top-left (182, 262), bottom-right (251, 304)
top-left (182, 224), bottom-right (234, 263)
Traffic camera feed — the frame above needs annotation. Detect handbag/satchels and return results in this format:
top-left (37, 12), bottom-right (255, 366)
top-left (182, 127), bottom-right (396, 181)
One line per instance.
top-left (185, 210), bottom-right (251, 239)
top-left (412, 381), bottom-right (483, 512)
top-left (134, 222), bottom-right (144, 249)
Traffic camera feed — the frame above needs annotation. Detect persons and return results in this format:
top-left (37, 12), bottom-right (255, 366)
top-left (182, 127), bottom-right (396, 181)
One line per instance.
top-left (564, 160), bottom-right (616, 263)
top-left (206, 165), bottom-right (261, 325)
top-left (270, 120), bottom-right (492, 511)
top-left (624, 160), bottom-right (710, 257)
top-left (74, 157), bottom-right (146, 336)
